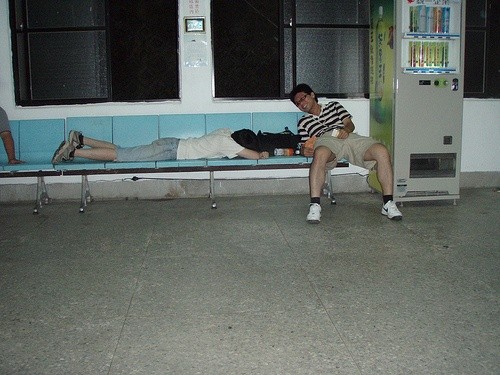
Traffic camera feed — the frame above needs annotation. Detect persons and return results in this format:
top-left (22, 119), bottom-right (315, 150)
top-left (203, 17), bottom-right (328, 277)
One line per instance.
top-left (52, 129), bottom-right (269, 166)
top-left (0, 107), bottom-right (26, 164)
top-left (288, 83), bottom-right (403, 224)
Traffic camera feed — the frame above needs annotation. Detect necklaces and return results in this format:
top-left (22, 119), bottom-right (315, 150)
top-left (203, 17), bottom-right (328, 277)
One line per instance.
top-left (311, 109), bottom-right (329, 127)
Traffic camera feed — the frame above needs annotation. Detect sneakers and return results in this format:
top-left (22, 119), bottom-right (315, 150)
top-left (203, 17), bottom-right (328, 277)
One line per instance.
top-left (52, 141), bottom-right (74, 164)
top-left (69, 130), bottom-right (84, 149)
top-left (306, 203), bottom-right (321, 223)
top-left (381, 200), bottom-right (402, 220)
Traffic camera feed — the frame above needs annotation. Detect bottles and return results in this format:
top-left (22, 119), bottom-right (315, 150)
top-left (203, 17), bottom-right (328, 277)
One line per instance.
top-left (409, 0), bottom-right (451, 33)
top-left (374, 6), bottom-right (386, 124)
top-left (274, 148), bottom-right (300, 156)
top-left (408, 42), bottom-right (449, 68)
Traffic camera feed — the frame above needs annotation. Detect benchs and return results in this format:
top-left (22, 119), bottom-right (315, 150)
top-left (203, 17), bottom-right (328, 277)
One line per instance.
top-left (0, 118), bottom-right (65, 216)
top-left (55, 111), bottom-right (349, 214)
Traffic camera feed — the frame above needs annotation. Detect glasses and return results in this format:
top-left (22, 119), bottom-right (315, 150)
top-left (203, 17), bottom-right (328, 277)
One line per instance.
top-left (296, 94), bottom-right (308, 107)
